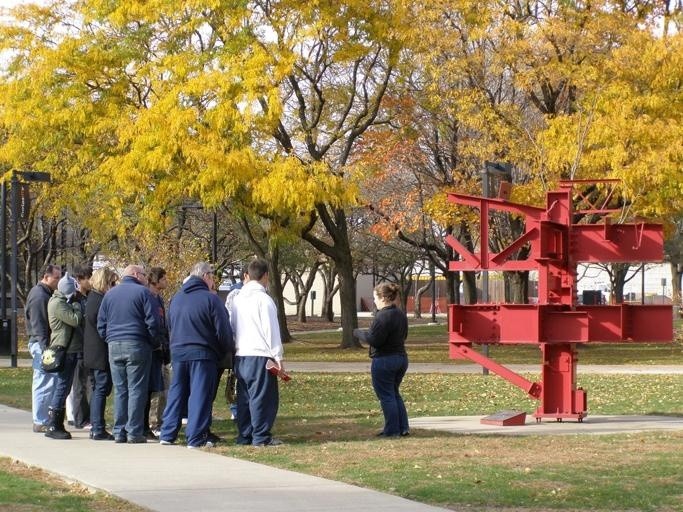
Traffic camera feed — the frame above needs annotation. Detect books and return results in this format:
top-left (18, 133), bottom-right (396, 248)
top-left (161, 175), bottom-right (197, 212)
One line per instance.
top-left (265, 359), bottom-right (291, 383)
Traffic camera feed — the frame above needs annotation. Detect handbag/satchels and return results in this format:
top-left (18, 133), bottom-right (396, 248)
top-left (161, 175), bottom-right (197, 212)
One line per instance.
top-left (42, 345), bottom-right (65, 373)
top-left (226, 375), bottom-right (239, 404)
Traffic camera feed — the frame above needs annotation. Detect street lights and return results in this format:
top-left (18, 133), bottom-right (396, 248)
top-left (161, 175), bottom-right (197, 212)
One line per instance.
top-left (7, 169), bottom-right (52, 367)
top-left (477, 155), bottom-right (510, 376)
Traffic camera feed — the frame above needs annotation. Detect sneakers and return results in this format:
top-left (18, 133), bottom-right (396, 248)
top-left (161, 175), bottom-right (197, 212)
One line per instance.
top-left (378, 431), bottom-right (410, 437)
top-left (32, 414), bottom-right (282, 449)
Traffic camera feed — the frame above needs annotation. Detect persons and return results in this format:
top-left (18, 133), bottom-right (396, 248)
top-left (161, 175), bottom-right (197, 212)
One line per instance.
top-left (352, 283), bottom-right (409, 437)
top-left (96, 265), bottom-right (160, 443)
top-left (24, 264), bottom-right (75, 432)
top-left (229, 257), bottom-right (285, 447)
top-left (84, 266), bottom-right (119, 441)
top-left (142, 267), bottom-right (170, 442)
top-left (224, 261), bottom-right (253, 420)
top-left (70, 265), bottom-right (95, 430)
top-left (41, 270), bottom-right (82, 438)
top-left (159, 261), bottom-right (233, 448)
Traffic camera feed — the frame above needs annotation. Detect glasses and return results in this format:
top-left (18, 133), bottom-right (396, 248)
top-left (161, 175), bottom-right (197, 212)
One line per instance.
top-left (151, 280), bottom-right (159, 285)
top-left (108, 281), bottom-right (116, 287)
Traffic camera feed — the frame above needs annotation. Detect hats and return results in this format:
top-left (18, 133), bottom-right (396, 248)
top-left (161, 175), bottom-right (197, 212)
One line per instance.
top-left (57, 272), bottom-right (79, 303)
